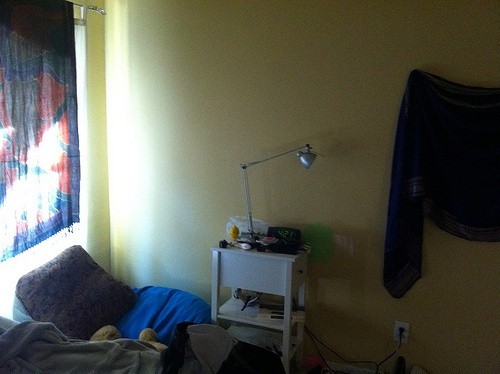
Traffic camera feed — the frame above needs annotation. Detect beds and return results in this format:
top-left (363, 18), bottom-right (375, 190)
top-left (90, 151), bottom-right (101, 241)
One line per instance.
top-left (0, 287), bottom-right (211, 374)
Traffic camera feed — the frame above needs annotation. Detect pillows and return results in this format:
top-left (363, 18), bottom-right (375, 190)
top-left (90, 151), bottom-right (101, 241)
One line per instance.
top-left (16, 243), bottom-right (137, 340)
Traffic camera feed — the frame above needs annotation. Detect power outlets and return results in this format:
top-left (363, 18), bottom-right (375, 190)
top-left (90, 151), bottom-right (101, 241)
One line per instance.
top-left (393, 321), bottom-right (411, 343)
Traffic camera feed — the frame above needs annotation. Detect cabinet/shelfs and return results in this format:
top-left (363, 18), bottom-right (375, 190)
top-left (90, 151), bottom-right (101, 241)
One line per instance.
top-left (209, 244), bottom-right (310, 374)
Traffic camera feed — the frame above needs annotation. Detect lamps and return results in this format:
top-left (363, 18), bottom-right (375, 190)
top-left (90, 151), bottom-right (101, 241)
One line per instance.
top-left (241, 144), bottom-right (317, 242)
